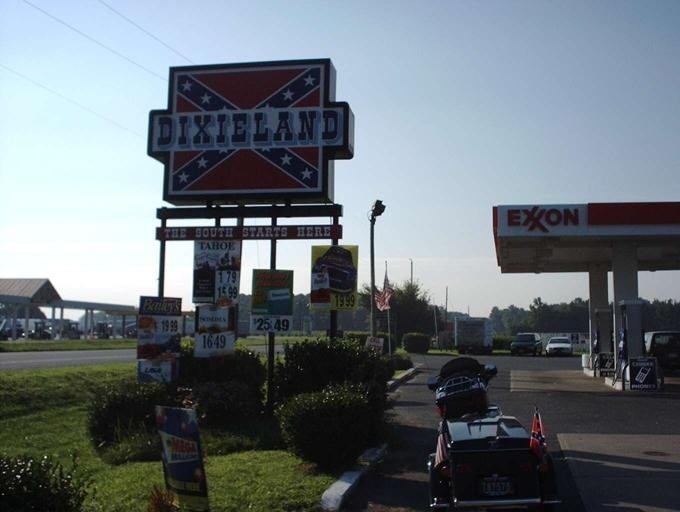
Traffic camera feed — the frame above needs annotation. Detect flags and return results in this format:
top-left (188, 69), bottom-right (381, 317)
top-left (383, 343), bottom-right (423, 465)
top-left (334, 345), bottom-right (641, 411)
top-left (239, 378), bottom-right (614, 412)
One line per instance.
top-left (529, 411), bottom-right (548, 460)
top-left (379, 271), bottom-right (394, 310)
top-left (374, 285), bottom-right (390, 312)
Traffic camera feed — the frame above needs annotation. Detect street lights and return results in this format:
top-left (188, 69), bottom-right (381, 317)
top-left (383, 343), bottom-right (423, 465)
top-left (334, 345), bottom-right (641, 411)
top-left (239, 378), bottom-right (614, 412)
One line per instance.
top-left (369, 199), bottom-right (386, 336)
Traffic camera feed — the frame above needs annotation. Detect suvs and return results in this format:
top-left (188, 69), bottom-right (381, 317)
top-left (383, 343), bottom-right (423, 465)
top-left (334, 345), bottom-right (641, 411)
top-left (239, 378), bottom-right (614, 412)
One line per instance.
top-left (510, 332), bottom-right (543, 358)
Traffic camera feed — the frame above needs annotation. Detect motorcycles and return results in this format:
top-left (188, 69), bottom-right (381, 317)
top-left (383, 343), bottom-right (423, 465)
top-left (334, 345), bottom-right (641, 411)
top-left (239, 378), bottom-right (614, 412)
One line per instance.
top-left (424, 357), bottom-right (560, 511)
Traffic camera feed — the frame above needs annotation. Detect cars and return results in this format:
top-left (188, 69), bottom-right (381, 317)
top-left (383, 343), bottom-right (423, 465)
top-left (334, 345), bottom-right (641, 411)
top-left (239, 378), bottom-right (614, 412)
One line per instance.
top-left (642, 330), bottom-right (680, 377)
top-left (545, 337), bottom-right (573, 356)
